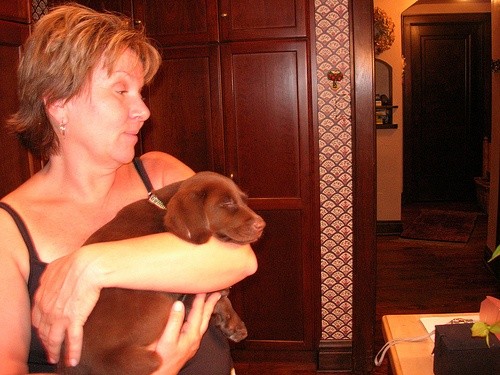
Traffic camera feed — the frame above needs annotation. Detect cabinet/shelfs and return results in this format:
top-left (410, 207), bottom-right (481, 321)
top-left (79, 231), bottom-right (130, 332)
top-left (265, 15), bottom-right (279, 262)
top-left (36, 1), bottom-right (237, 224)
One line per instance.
top-left (0, 0), bottom-right (320, 375)
top-left (375, 58), bottom-right (399, 129)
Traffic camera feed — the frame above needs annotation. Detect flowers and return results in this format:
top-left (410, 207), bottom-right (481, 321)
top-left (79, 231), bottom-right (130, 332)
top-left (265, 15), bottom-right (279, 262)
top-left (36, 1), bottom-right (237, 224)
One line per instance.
top-left (471, 295), bottom-right (500, 349)
top-left (374, 6), bottom-right (395, 56)
top-left (327, 68), bottom-right (343, 88)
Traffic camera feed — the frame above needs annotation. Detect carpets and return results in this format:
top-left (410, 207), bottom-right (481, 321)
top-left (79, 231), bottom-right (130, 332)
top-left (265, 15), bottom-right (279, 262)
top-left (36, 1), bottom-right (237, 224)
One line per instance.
top-left (399, 208), bottom-right (480, 244)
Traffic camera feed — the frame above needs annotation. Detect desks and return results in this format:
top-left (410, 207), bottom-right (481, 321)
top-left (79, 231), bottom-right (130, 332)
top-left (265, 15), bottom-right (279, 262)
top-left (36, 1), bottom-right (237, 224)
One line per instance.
top-left (381, 313), bottom-right (500, 375)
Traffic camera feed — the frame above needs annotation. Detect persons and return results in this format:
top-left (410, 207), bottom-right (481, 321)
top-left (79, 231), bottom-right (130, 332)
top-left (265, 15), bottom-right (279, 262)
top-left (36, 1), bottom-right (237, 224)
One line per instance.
top-left (0, 4), bottom-right (259, 375)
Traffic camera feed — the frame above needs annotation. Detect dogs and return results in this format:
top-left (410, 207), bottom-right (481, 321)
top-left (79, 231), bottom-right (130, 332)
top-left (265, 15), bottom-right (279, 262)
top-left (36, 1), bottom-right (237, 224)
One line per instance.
top-left (25, 171), bottom-right (267, 375)
